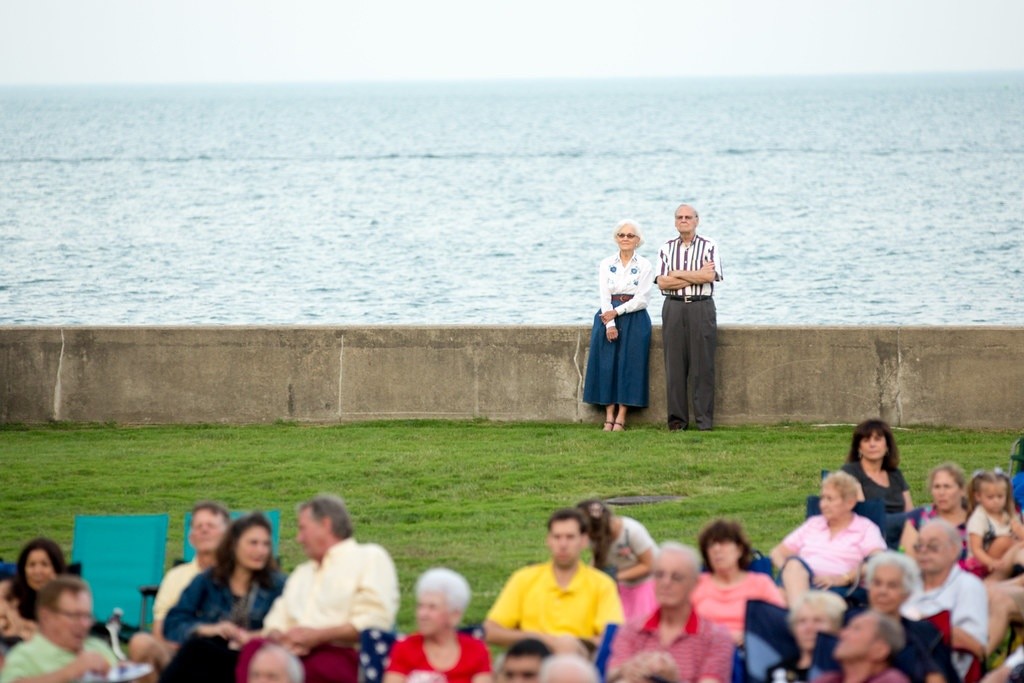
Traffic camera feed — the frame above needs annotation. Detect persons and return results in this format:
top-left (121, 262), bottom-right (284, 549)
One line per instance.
top-left (652, 204), bottom-right (723, 432)
top-left (0, 419), bottom-right (1024, 683)
top-left (583, 218), bottom-right (653, 431)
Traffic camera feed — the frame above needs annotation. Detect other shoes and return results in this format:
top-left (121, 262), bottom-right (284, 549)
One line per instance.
top-left (670, 421), bottom-right (682, 430)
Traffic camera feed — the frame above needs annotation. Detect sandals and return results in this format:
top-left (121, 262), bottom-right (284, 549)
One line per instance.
top-left (603, 422), bottom-right (614, 431)
top-left (612, 423), bottom-right (626, 432)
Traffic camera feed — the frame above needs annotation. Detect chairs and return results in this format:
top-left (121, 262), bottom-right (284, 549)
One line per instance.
top-left (0, 466), bottom-right (1024, 683)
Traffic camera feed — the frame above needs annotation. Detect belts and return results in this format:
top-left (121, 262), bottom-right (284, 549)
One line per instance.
top-left (670, 296), bottom-right (708, 303)
top-left (612, 295), bottom-right (632, 302)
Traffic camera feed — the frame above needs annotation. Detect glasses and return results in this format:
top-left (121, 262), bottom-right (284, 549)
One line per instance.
top-left (44, 606), bottom-right (92, 622)
top-left (655, 569), bottom-right (685, 584)
top-left (914, 542), bottom-right (944, 551)
top-left (617, 233), bottom-right (635, 239)
top-left (636, 670), bottom-right (669, 683)
